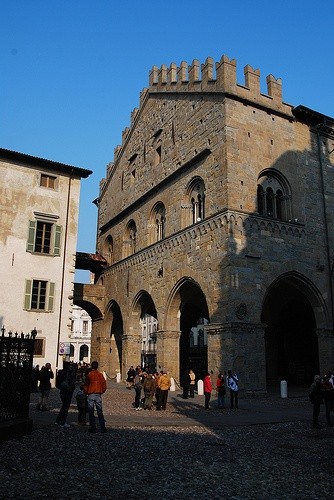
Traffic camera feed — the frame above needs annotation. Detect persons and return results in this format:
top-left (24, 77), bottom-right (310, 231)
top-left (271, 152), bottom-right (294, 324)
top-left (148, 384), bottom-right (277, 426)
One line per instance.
top-left (204, 373), bottom-right (212, 411)
top-left (84, 361), bottom-right (108, 432)
top-left (70, 361), bottom-right (89, 378)
top-left (135, 371), bottom-right (171, 411)
top-left (77, 384), bottom-right (90, 425)
top-left (127, 365), bottom-right (141, 389)
top-left (37, 363), bottom-right (54, 411)
top-left (54, 362), bottom-right (77, 427)
top-left (311, 371), bottom-right (334, 428)
top-left (32, 365), bottom-right (39, 393)
top-left (182, 370), bottom-right (191, 399)
top-left (226, 370), bottom-right (239, 409)
top-left (188, 370), bottom-right (195, 398)
top-left (217, 371), bottom-right (227, 409)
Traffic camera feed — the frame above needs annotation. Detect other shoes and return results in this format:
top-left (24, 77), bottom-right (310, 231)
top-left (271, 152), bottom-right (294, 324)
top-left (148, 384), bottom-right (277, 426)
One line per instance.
top-left (41, 407), bottom-right (48, 411)
top-left (156, 408), bottom-right (161, 410)
top-left (144, 407), bottom-right (147, 410)
top-left (38, 403), bottom-right (41, 410)
top-left (88, 428), bottom-right (97, 433)
top-left (148, 407), bottom-right (152, 410)
top-left (231, 405), bottom-right (233, 408)
top-left (235, 405), bottom-right (239, 408)
top-left (101, 427), bottom-right (108, 432)
top-left (54, 423), bottom-right (58, 425)
top-left (61, 424), bottom-right (70, 428)
top-left (315, 424), bottom-right (323, 429)
